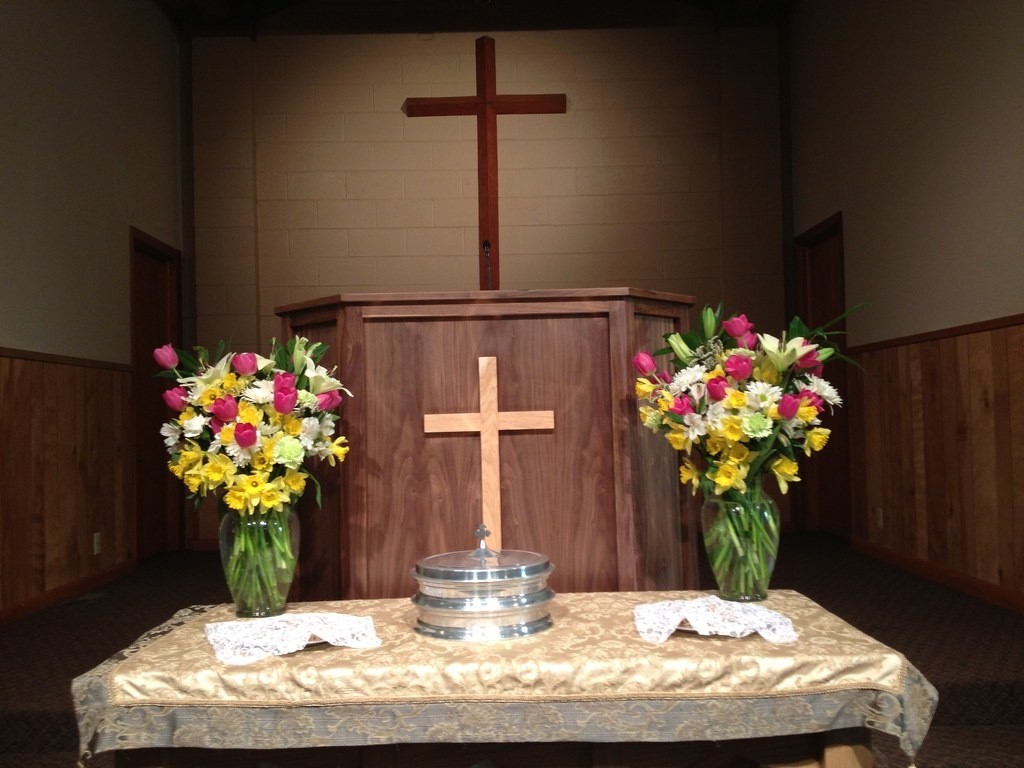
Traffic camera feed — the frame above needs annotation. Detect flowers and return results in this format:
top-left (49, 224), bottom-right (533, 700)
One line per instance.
top-left (632, 299), bottom-right (863, 597)
top-left (153, 328), bottom-right (356, 614)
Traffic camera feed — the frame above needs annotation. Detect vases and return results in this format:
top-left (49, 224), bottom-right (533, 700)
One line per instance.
top-left (700, 476), bottom-right (781, 602)
top-left (219, 502), bottom-right (300, 617)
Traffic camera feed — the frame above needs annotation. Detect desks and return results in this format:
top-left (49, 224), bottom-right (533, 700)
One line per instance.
top-left (71, 588), bottom-right (939, 767)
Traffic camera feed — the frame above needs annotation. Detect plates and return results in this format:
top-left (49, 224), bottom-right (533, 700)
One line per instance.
top-left (676, 626), bottom-right (695, 631)
top-left (307, 640), bottom-right (326, 645)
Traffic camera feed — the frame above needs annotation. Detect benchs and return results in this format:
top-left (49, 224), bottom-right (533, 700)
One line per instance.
top-left (0, 550), bottom-right (229, 768)
top-left (772, 532), bottom-right (1024, 768)
